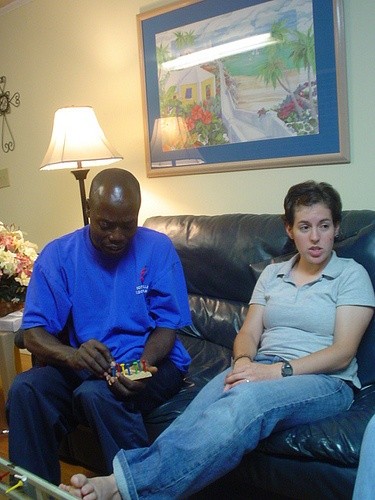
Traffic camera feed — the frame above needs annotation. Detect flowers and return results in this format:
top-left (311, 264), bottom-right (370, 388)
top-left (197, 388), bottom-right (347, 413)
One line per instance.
top-left (0, 220), bottom-right (39, 304)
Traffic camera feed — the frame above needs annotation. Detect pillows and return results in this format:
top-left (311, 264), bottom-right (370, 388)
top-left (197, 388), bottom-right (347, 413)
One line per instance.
top-left (247, 220), bottom-right (375, 390)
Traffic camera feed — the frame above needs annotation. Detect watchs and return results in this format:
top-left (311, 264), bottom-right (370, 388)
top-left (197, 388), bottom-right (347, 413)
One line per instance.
top-left (279, 358), bottom-right (294, 377)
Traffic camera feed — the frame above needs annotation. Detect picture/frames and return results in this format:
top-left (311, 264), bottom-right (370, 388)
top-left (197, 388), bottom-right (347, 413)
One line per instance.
top-left (136, 0), bottom-right (352, 180)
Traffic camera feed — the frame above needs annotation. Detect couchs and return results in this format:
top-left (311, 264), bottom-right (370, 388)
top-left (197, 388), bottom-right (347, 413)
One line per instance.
top-left (12, 209), bottom-right (375, 500)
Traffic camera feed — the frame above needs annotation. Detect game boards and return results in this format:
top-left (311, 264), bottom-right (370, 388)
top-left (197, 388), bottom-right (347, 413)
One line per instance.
top-left (108, 367), bottom-right (152, 381)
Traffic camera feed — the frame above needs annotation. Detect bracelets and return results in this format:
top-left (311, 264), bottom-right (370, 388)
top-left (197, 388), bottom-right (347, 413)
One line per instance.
top-left (231, 353), bottom-right (254, 364)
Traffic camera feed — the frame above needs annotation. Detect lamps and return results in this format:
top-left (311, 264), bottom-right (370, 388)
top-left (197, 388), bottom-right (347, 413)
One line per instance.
top-left (149, 115), bottom-right (206, 166)
top-left (39, 104), bottom-right (125, 229)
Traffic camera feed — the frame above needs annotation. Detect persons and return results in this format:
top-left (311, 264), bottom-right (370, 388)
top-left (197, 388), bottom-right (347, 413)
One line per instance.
top-left (6, 168), bottom-right (194, 486)
top-left (57, 179), bottom-right (375, 500)
